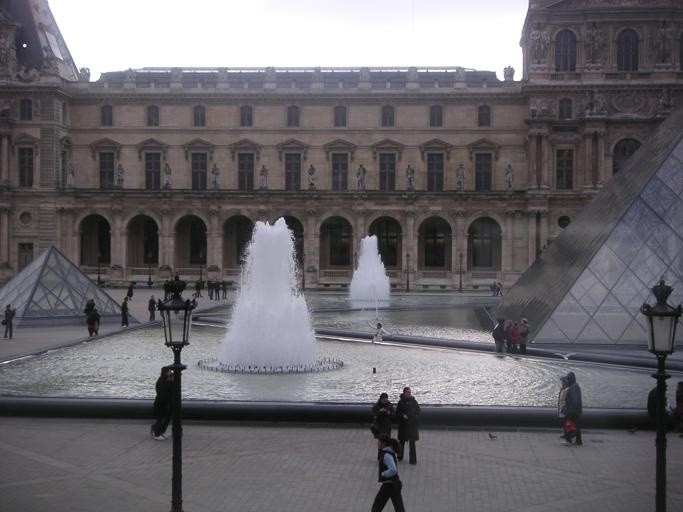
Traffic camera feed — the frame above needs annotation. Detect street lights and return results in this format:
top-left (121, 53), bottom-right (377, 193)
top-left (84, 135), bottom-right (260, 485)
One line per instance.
top-left (299, 252), bottom-right (306, 291)
top-left (457, 252), bottom-right (463, 292)
top-left (639, 271), bottom-right (682, 512)
top-left (150, 291), bottom-right (199, 512)
top-left (404, 252), bottom-right (412, 293)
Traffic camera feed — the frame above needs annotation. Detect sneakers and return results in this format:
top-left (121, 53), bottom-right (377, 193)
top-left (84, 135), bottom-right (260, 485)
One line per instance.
top-left (150, 426), bottom-right (167, 440)
top-left (575, 441), bottom-right (582, 445)
top-left (561, 442), bottom-right (573, 446)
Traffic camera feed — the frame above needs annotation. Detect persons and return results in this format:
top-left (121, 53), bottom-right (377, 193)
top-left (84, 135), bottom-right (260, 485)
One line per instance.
top-left (405, 163), bottom-right (415, 190)
top-left (305, 163), bottom-right (317, 188)
top-left (369, 392), bottom-right (395, 459)
top-left (363, 320), bottom-right (391, 344)
top-left (114, 161), bottom-right (124, 190)
top-left (1, 269), bottom-right (233, 341)
top-left (491, 314), bottom-right (531, 354)
top-left (160, 163), bottom-right (173, 191)
top-left (257, 164), bottom-right (270, 191)
top-left (453, 163), bottom-right (465, 192)
top-left (490, 281), bottom-right (503, 298)
top-left (353, 162), bottom-right (367, 190)
top-left (394, 386), bottom-right (421, 466)
top-left (208, 162), bottom-right (222, 191)
top-left (370, 431), bottom-right (406, 512)
top-left (558, 377), bottom-right (569, 441)
top-left (562, 370), bottom-right (584, 445)
top-left (502, 163), bottom-right (515, 189)
